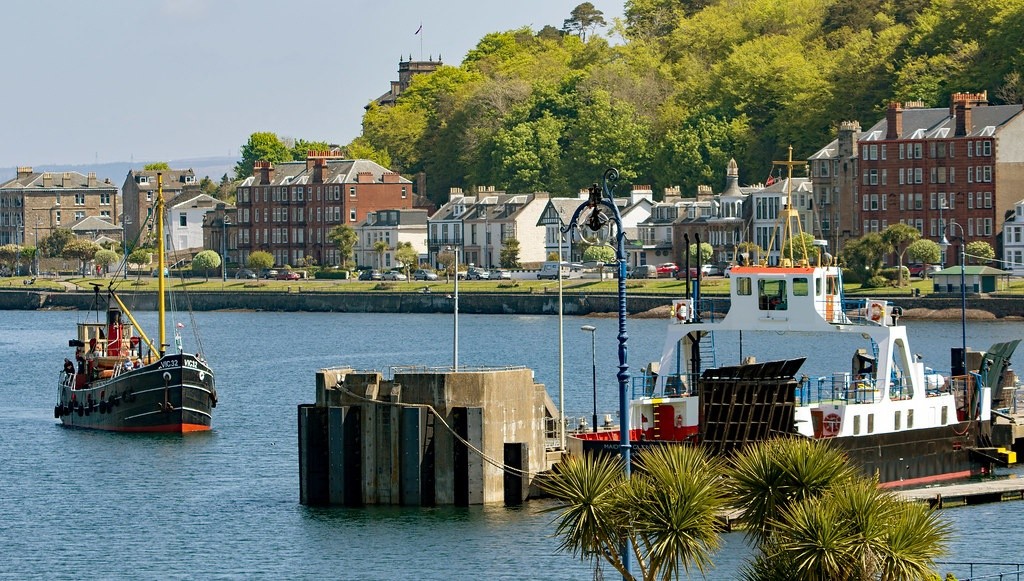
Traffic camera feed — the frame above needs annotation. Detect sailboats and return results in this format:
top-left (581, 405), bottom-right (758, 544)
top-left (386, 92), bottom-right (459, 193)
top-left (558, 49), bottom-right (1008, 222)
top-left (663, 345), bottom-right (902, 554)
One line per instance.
top-left (54, 170), bottom-right (217, 434)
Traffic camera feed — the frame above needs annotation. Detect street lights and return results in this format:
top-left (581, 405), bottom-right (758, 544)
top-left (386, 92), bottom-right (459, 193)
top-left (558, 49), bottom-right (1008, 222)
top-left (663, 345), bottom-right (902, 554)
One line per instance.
top-left (577, 324), bottom-right (599, 433)
top-left (936, 221), bottom-right (968, 409)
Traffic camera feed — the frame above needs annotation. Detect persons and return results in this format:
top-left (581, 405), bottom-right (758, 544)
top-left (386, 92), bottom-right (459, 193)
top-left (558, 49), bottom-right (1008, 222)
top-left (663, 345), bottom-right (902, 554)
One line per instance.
top-left (124, 356), bottom-right (143, 371)
top-left (62, 357), bottom-right (95, 386)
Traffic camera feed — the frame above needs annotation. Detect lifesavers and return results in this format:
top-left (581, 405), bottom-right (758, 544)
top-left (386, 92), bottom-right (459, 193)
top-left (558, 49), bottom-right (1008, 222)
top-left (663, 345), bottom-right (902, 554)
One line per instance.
top-left (866, 303), bottom-right (883, 321)
top-left (823, 413), bottom-right (842, 432)
top-left (676, 302), bottom-right (692, 320)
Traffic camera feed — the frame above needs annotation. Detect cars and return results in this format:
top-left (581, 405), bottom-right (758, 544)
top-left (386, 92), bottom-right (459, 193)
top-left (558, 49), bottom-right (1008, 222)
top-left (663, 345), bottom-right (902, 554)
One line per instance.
top-left (358, 269), bottom-right (383, 280)
top-left (258, 266), bottom-right (279, 280)
top-left (656, 263), bottom-right (678, 278)
top-left (701, 264), bottom-right (721, 277)
top-left (723, 264), bottom-right (740, 278)
top-left (274, 270), bottom-right (301, 281)
top-left (153, 266), bottom-right (168, 277)
top-left (626, 266), bottom-right (637, 279)
top-left (891, 266), bottom-right (911, 278)
top-left (76, 266), bottom-right (90, 276)
top-left (381, 271), bottom-right (406, 281)
top-left (675, 268), bottom-right (703, 280)
top-left (488, 269), bottom-right (511, 280)
top-left (630, 264), bottom-right (659, 280)
top-left (909, 262), bottom-right (935, 278)
top-left (235, 269), bottom-right (257, 280)
top-left (412, 269), bottom-right (438, 281)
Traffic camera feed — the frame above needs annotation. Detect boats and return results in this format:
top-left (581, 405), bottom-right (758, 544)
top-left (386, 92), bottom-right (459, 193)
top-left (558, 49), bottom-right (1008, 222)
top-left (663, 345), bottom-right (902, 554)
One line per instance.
top-left (572, 143), bottom-right (962, 473)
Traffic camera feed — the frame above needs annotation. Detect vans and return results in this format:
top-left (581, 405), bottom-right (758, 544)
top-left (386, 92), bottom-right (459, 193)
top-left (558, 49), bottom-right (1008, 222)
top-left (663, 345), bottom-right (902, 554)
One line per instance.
top-left (467, 266), bottom-right (490, 279)
top-left (536, 261), bottom-right (571, 279)
